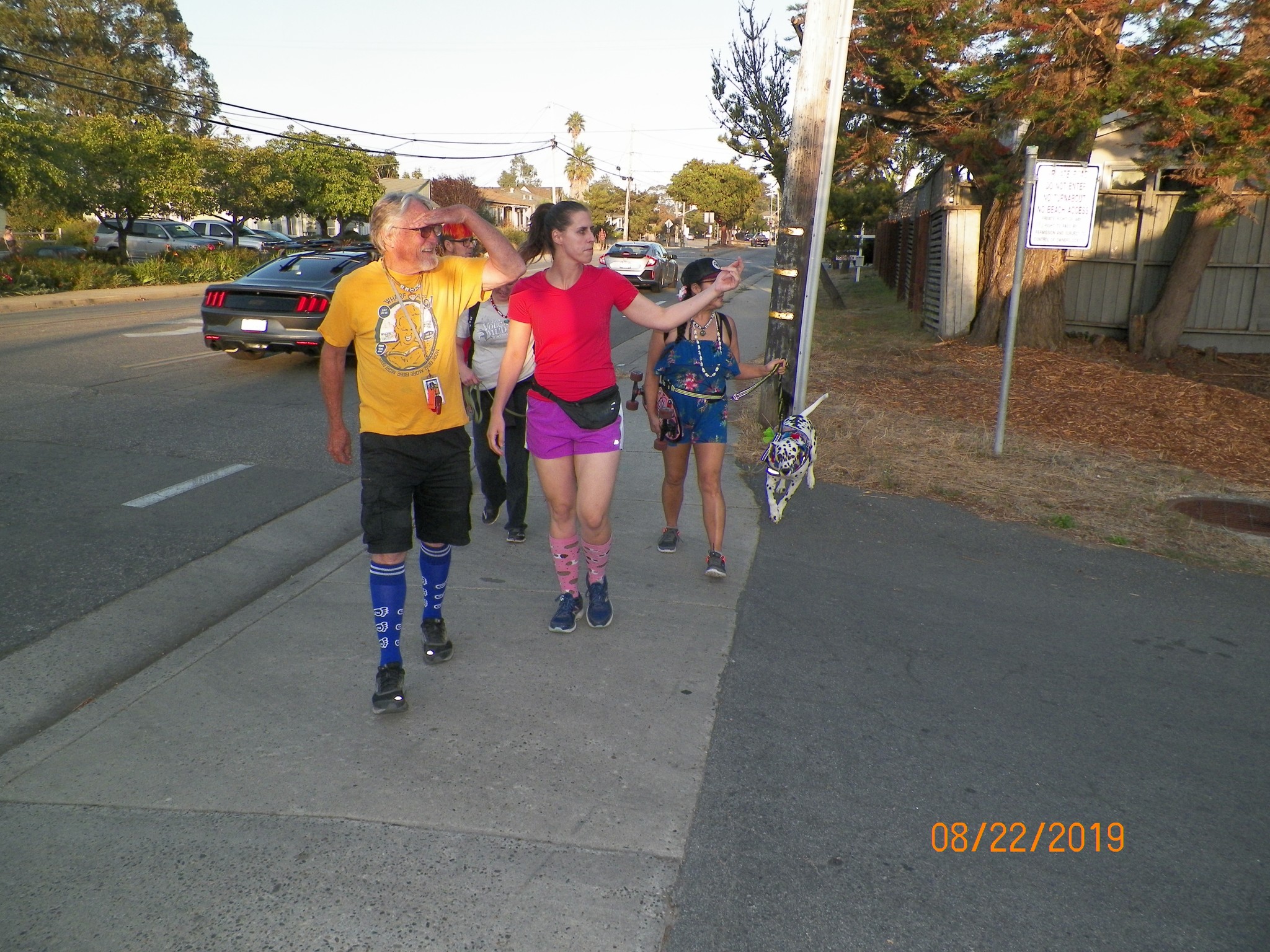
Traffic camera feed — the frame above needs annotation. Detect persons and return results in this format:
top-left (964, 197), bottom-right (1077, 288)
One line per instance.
top-left (317, 191), bottom-right (527, 715)
top-left (447, 202), bottom-right (786, 631)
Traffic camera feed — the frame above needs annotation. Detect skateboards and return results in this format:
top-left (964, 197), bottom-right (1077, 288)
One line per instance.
top-left (625, 370), bottom-right (683, 452)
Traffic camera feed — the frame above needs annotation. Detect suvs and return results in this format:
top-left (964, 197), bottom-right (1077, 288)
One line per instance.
top-left (92, 217), bottom-right (228, 266)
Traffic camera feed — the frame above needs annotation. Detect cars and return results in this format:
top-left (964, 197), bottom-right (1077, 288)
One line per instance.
top-left (18, 243), bottom-right (89, 263)
top-left (201, 242), bottom-right (382, 367)
top-left (251, 228), bottom-right (298, 245)
top-left (751, 235), bottom-right (768, 247)
top-left (598, 241), bottom-right (679, 293)
top-left (744, 233), bottom-right (753, 241)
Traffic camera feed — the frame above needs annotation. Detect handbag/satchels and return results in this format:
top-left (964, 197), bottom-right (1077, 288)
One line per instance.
top-left (557, 384), bottom-right (622, 429)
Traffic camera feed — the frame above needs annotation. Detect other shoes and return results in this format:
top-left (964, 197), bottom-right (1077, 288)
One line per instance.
top-left (507, 527), bottom-right (527, 543)
top-left (482, 505), bottom-right (500, 525)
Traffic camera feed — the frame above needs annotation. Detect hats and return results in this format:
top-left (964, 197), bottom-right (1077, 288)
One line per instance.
top-left (681, 258), bottom-right (722, 287)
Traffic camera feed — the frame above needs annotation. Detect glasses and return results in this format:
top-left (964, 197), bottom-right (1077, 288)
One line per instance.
top-left (392, 224), bottom-right (443, 239)
top-left (451, 238), bottom-right (478, 247)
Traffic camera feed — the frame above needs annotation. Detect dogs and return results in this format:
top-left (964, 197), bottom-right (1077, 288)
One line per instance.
top-left (765, 393), bottom-right (828, 523)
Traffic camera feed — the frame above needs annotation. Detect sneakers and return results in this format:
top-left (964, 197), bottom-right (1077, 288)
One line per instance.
top-left (585, 570), bottom-right (613, 627)
top-left (548, 591), bottom-right (583, 633)
top-left (371, 659), bottom-right (409, 714)
top-left (420, 617), bottom-right (453, 665)
top-left (704, 549), bottom-right (727, 577)
top-left (657, 526), bottom-right (684, 553)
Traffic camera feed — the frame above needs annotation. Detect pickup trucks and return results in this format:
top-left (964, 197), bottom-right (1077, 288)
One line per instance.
top-left (190, 220), bottom-right (288, 258)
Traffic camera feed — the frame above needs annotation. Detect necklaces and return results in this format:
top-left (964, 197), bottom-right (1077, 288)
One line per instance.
top-left (690, 310), bottom-right (715, 336)
top-left (692, 313), bottom-right (722, 378)
top-left (490, 295), bottom-right (510, 323)
top-left (382, 254), bottom-right (424, 301)
